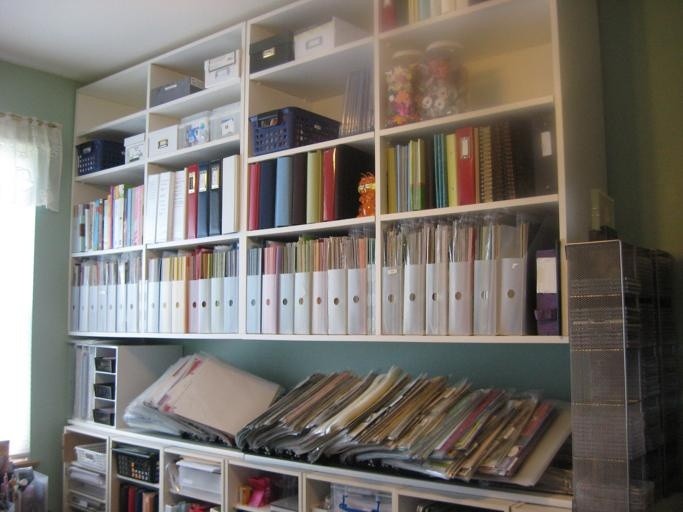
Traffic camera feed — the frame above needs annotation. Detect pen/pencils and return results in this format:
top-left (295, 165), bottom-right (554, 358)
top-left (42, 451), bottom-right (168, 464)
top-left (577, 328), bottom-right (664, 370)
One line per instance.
top-left (0, 474), bottom-right (34, 512)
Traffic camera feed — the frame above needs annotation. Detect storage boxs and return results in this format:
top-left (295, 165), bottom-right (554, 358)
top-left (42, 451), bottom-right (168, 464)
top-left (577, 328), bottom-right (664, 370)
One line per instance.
top-left (75, 140), bottom-right (125, 175)
top-left (73, 442), bottom-right (108, 472)
top-left (147, 122), bottom-right (181, 159)
top-left (176, 466), bottom-right (220, 504)
top-left (330, 482), bottom-right (392, 512)
top-left (94, 357), bottom-right (114, 374)
top-left (179, 109), bottom-right (209, 150)
top-left (204, 48), bottom-right (240, 89)
top-left (209, 100), bottom-right (239, 143)
top-left (94, 382), bottom-right (114, 399)
top-left (293, 16), bottom-right (371, 60)
top-left (250, 30), bottom-right (293, 74)
top-left (150, 75), bottom-right (205, 109)
top-left (92, 405), bottom-right (113, 426)
top-left (248, 106), bottom-right (341, 157)
top-left (110, 451), bottom-right (159, 484)
top-left (124, 132), bottom-right (144, 164)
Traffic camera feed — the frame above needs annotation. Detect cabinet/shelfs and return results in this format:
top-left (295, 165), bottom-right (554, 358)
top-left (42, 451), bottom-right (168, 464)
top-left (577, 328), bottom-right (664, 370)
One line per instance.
top-left (163, 447), bottom-right (225, 512)
top-left (61, 424), bottom-right (108, 512)
top-left (66, 341), bottom-right (184, 429)
top-left (395, 485), bottom-right (521, 512)
top-left (224, 459), bottom-right (312, 511)
top-left (108, 435), bottom-right (173, 512)
top-left (303, 470), bottom-right (409, 512)
top-left (564, 238), bottom-right (682, 512)
top-left (510, 502), bottom-right (570, 511)
top-left (247, 0), bottom-right (610, 343)
top-left (66, 21), bottom-right (246, 338)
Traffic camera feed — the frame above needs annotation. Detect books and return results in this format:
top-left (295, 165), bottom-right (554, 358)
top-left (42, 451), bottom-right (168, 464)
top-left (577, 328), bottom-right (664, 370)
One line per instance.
top-left (386, 120), bottom-right (529, 213)
top-left (70, 253), bottom-right (144, 335)
top-left (148, 242), bottom-right (239, 333)
top-left (381, 212), bottom-right (531, 336)
top-left (248, 142), bottom-right (371, 231)
top-left (145, 174), bottom-right (157, 244)
top-left (71, 182), bottom-right (144, 255)
top-left (246, 224), bottom-right (377, 336)
top-left (122, 349), bottom-right (286, 449)
top-left (237, 363), bottom-right (574, 490)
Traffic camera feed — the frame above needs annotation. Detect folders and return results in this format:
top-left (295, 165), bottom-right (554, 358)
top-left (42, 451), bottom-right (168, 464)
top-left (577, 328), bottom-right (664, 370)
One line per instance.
top-left (310, 237), bottom-right (328, 336)
top-left (211, 245), bottom-right (225, 334)
top-left (448, 214), bottom-right (473, 337)
top-left (197, 251), bottom-right (212, 333)
top-left (347, 228), bottom-right (367, 335)
top-left (70, 258), bottom-right (80, 333)
top-left (172, 249), bottom-right (190, 335)
top-left (116, 256), bottom-right (127, 334)
top-left (454, 126), bottom-right (477, 208)
top-left (159, 252), bottom-right (172, 334)
top-left (106, 258), bottom-right (116, 333)
top-left (260, 240), bottom-right (279, 337)
top-left (223, 244), bottom-right (240, 335)
top-left (425, 220), bottom-right (449, 337)
top-left (473, 214), bottom-right (498, 338)
top-left (88, 261), bottom-right (98, 332)
top-left (294, 239), bottom-right (312, 335)
top-left (127, 253), bottom-right (139, 334)
top-left (402, 223), bottom-right (425, 337)
top-left (148, 250), bottom-right (160, 334)
top-left (143, 174), bottom-right (159, 246)
top-left (79, 257), bottom-right (89, 333)
top-left (187, 247), bottom-right (200, 334)
top-left (325, 235), bottom-right (348, 336)
top-left (247, 237), bottom-right (263, 335)
top-left (277, 240), bottom-right (296, 335)
top-left (97, 257), bottom-right (108, 334)
top-left (382, 213), bottom-right (404, 336)
top-left (494, 213), bottom-right (528, 337)
